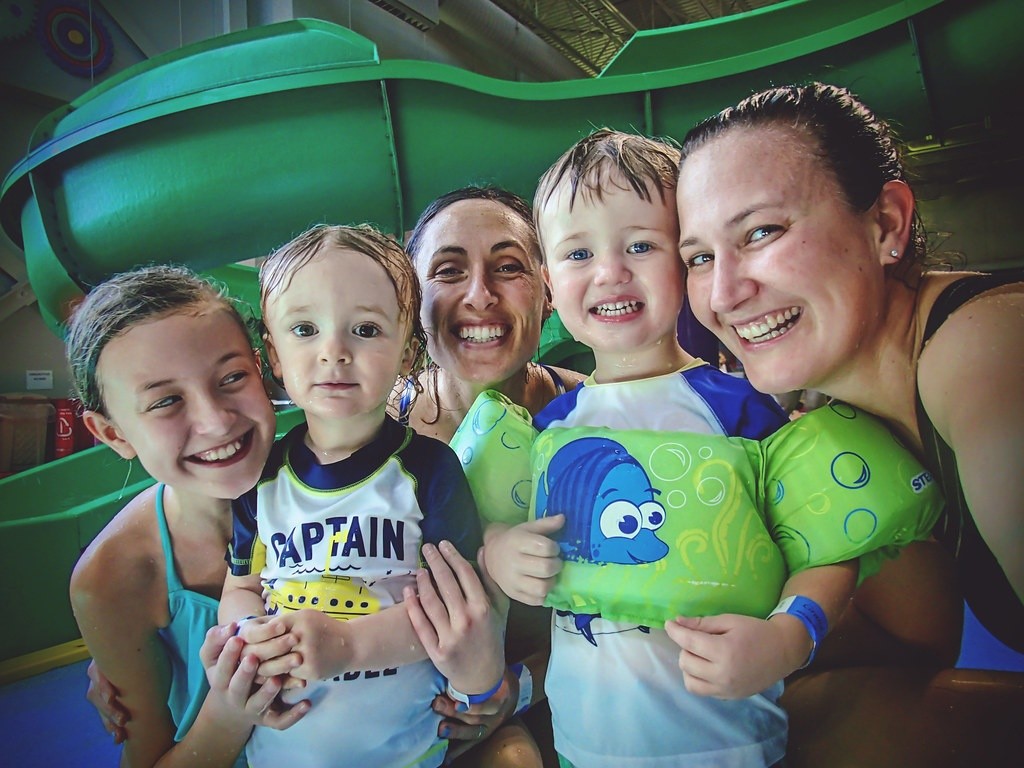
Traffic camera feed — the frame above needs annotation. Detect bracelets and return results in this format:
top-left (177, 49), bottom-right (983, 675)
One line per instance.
top-left (765, 595), bottom-right (828, 669)
top-left (447, 675), bottom-right (504, 709)
top-left (234, 615), bottom-right (257, 636)
top-left (508, 662), bottom-right (533, 718)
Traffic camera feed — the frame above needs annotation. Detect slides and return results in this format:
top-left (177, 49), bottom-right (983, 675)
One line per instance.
top-left (2, 0), bottom-right (1023, 676)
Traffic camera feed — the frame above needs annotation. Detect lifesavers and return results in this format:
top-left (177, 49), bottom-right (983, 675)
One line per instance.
top-left (435, 381), bottom-right (943, 631)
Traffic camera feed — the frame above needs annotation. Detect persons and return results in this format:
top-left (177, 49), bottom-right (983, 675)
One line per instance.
top-left (481, 126), bottom-right (861, 768)
top-left (217, 224), bottom-right (486, 768)
top-left (67, 267), bottom-right (507, 768)
top-left (86, 186), bottom-right (591, 768)
top-left (675, 82), bottom-right (1024, 768)
